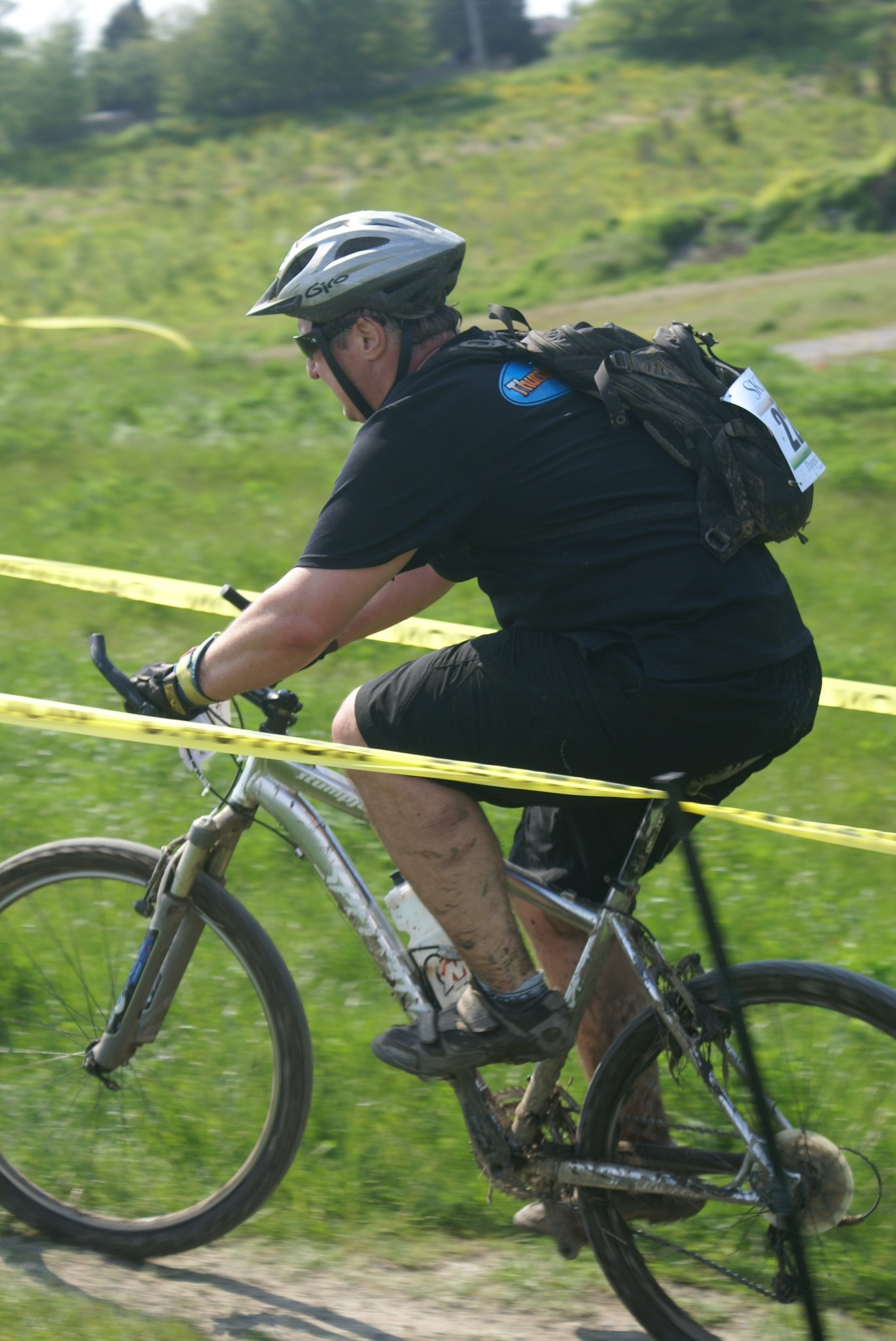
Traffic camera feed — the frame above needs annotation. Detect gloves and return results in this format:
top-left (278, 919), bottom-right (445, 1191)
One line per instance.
top-left (123, 631), bottom-right (219, 722)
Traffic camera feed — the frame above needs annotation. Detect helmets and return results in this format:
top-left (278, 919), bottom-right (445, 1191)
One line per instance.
top-left (245, 209), bottom-right (486, 323)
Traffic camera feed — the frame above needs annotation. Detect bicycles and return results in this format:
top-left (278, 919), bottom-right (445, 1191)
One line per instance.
top-left (1, 582), bottom-right (895, 1341)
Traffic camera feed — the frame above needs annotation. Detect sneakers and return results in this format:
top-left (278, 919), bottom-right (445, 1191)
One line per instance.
top-left (370, 977), bottom-right (580, 1080)
top-left (510, 1141), bottom-right (708, 1234)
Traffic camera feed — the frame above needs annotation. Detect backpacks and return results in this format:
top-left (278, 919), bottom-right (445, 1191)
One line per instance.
top-left (423, 305), bottom-right (824, 554)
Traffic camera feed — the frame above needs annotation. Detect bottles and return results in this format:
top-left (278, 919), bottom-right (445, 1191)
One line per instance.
top-left (385, 870), bottom-right (474, 1009)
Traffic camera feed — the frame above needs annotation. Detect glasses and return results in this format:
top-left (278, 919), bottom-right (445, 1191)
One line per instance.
top-left (292, 324), bottom-right (348, 359)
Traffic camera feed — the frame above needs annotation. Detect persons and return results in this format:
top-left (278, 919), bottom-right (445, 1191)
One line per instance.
top-left (139, 211), bottom-right (822, 1242)
